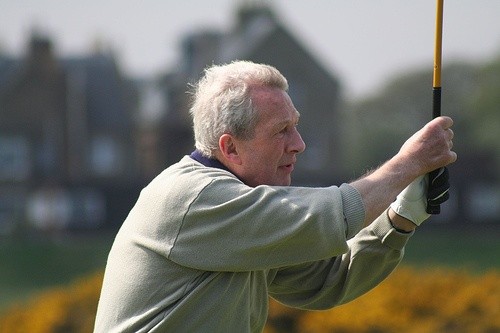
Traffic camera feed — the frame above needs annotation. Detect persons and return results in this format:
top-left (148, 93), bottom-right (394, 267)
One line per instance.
top-left (91, 59), bottom-right (459, 330)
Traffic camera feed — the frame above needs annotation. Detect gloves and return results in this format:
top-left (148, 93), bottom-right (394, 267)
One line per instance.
top-left (391, 164), bottom-right (451, 226)
